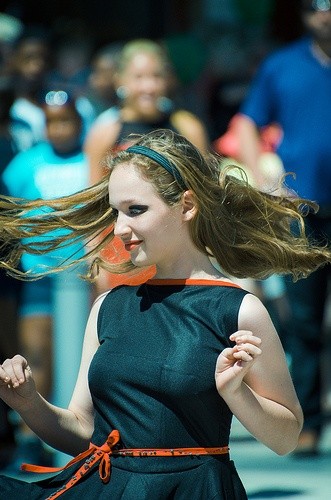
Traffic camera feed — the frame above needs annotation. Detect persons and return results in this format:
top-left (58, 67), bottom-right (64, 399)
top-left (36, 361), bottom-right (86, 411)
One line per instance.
top-left (0, 28), bottom-right (293, 462)
top-left (0, 128), bottom-right (304, 500)
top-left (221, 1), bottom-right (331, 457)
